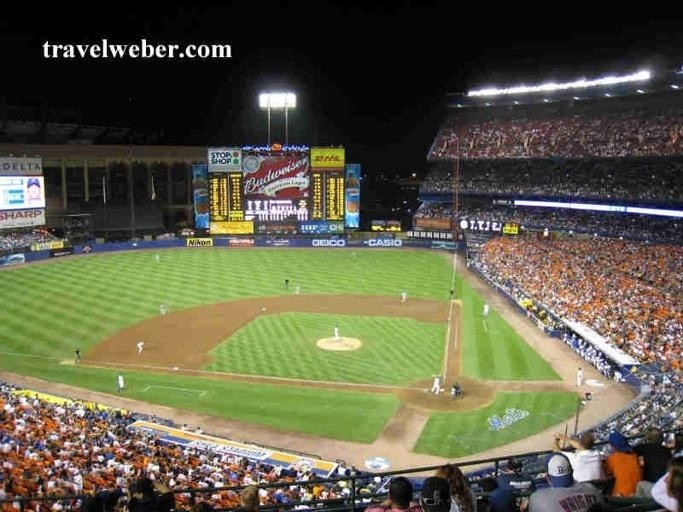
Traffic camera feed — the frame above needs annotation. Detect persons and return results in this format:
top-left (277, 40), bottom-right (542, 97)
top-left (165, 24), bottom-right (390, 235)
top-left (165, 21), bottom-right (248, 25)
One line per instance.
top-left (1, 111), bottom-right (683, 512)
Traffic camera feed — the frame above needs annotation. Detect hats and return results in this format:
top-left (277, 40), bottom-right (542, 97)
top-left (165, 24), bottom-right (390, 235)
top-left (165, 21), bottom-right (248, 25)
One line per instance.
top-left (609, 433), bottom-right (632, 453)
top-left (545, 452), bottom-right (574, 488)
top-left (420, 476), bottom-right (452, 512)
